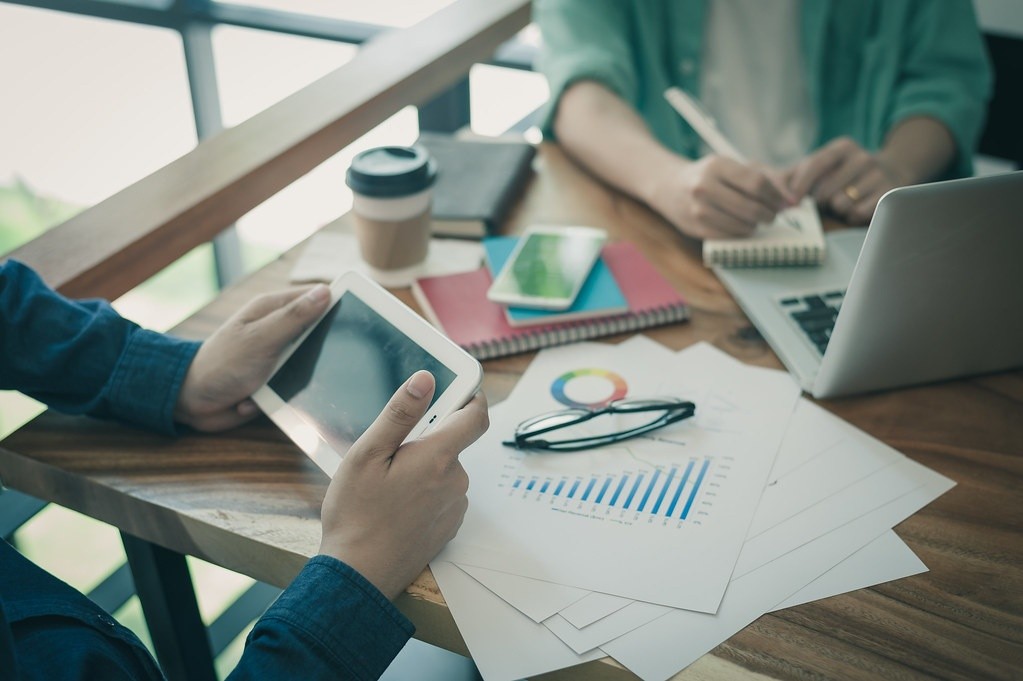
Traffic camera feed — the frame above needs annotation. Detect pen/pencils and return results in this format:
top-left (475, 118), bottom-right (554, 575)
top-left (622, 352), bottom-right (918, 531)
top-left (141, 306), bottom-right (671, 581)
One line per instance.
top-left (663, 87), bottom-right (804, 232)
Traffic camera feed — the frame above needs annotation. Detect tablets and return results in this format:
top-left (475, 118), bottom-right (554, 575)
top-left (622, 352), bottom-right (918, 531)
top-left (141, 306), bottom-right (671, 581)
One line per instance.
top-left (251, 268), bottom-right (484, 479)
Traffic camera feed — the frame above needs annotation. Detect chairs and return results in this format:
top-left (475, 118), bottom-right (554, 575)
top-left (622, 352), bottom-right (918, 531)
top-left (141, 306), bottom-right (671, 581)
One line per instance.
top-left (969, 25), bottom-right (1023, 189)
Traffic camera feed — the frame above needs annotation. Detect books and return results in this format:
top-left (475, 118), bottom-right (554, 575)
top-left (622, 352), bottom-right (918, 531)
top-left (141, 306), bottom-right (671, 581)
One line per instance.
top-left (703, 163), bottom-right (827, 271)
top-left (406, 243), bottom-right (693, 362)
top-left (409, 126), bottom-right (536, 239)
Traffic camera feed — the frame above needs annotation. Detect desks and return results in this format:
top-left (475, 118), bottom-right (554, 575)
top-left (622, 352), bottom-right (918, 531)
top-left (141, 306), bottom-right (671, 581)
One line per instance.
top-left (0, 126), bottom-right (1023, 681)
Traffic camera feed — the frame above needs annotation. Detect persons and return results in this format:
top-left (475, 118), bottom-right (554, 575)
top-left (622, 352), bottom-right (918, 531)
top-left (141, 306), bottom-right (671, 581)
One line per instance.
top-left (535, 0), bottom-right (992, 240)
top-left (0, 255), bottom-right (491, 681)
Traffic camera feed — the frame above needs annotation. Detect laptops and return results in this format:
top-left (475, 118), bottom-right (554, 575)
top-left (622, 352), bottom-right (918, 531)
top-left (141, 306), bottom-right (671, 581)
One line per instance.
top-left (712, 170), bottom-right (1023, 397)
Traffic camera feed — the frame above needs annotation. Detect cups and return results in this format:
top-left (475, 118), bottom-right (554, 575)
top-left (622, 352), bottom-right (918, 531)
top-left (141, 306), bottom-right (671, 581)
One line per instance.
top-left (346, 144), bottom-right (441, 284)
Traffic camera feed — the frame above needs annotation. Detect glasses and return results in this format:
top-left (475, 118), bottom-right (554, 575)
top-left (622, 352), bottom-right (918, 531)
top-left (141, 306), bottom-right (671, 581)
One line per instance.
top-left (502, 393), bottom-right (696, 452)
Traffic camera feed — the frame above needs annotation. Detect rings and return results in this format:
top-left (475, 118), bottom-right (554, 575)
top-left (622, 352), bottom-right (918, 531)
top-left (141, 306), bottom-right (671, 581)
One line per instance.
top-left (846, 185), bottom-right (861, 202)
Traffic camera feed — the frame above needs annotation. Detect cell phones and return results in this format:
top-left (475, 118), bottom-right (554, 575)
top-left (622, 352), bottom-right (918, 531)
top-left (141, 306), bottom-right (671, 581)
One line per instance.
top-left (487, 224), bottom-right (607, 313)
